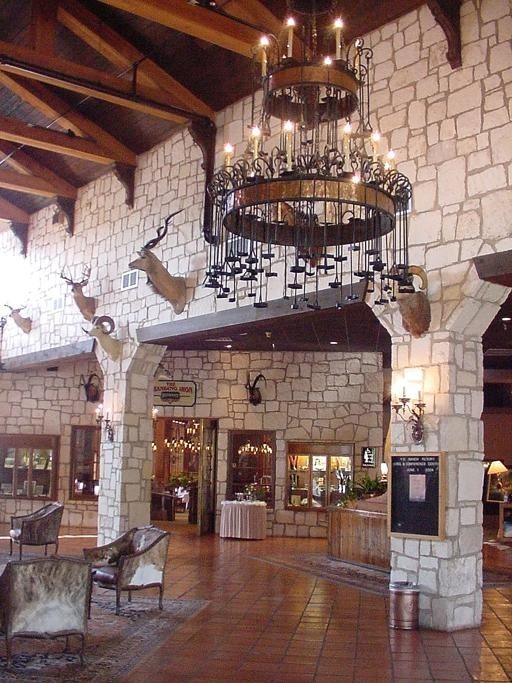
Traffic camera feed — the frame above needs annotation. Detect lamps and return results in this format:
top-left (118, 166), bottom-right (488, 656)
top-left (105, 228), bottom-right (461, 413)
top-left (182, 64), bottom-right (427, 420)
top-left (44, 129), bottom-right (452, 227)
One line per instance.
top-left (487, 461), bottom-right (508, 502)
top-left (203, 0), bottom-right (416, 310)
top-left (151, 419), bottom-right (272, 456)
top-left (96, 405), bottom-right (113, 442)
top-left (390, 385), bottom-right (425, 445)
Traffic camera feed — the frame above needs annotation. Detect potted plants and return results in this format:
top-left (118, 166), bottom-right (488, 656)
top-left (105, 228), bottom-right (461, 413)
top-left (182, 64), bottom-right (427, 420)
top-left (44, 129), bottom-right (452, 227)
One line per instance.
top-left (357, 477), bottom-right (386, 498)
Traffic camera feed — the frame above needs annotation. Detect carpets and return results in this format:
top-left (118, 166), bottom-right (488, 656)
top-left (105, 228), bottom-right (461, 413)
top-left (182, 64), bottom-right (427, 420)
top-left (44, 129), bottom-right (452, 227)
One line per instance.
top-left (242, 552), bottom-right (512, 598)
top-left (0, 594), bottom-right (213, 683)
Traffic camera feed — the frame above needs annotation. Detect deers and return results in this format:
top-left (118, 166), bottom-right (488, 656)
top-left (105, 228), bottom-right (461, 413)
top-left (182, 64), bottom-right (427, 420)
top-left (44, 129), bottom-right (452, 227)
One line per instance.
top-left (60, 261), bottom-right (96, 321)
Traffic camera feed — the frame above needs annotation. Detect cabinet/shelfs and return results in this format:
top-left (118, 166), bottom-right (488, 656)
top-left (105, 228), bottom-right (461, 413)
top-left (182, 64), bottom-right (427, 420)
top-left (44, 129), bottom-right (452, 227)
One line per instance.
top-left (289, 468), bottom-right (351, 491)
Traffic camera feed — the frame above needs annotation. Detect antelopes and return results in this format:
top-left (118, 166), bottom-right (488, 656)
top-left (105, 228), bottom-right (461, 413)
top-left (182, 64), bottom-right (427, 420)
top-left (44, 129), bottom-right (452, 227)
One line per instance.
top-left (128, 208), bottom-right (185, 305)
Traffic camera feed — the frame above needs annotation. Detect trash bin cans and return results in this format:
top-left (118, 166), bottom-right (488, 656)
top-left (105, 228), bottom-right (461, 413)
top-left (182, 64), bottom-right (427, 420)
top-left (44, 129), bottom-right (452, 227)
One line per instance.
top-left (389, 581), bottom-right (420, 631)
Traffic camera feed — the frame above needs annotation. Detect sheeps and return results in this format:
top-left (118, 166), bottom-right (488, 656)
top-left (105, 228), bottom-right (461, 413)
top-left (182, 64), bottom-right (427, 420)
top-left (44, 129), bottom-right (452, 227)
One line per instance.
top-left (79, 373), bottom-right (100, 402)
top-left (81, 316), bottom-right (121, 361)
top-left (245, 374), bottom-right (267, 406)
top-left (386, 265), bottom-right (431, 339)
top-left (4, 304), bottom-right (31, 334)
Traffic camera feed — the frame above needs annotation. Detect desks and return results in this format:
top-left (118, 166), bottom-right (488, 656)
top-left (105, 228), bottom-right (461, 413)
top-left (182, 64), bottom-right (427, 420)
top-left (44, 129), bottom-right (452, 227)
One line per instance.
top-left (220, 500), bottom-right (266, 540)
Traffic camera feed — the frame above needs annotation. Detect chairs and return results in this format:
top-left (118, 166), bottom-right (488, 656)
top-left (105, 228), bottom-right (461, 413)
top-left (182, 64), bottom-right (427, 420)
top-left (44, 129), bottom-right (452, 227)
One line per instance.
top-left (0, 553), bottom-right (90, 670)
top-left (10, 501), bottom-right (64, 561)
top-left (83, 527), bottom-right (172, 619)
